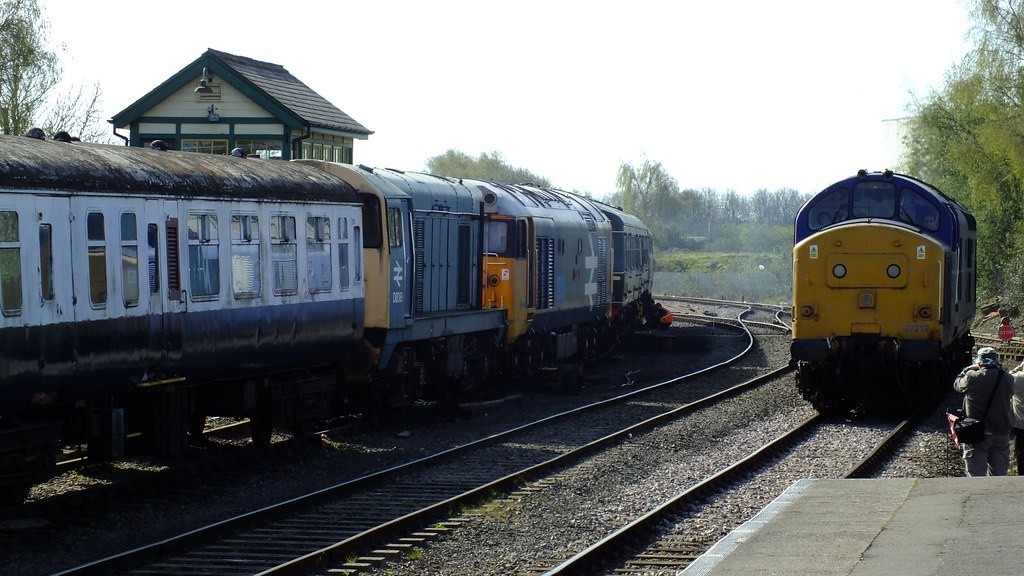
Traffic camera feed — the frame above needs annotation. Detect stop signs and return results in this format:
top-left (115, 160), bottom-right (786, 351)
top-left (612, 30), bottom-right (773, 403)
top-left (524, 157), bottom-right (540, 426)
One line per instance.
top-left (997, 324), bottom-right (1015, 341)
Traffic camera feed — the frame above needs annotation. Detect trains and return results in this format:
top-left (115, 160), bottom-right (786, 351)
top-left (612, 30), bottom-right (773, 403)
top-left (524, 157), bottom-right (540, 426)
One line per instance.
top-left (790, 168), bottom-right (980, 424)
top-left (0, 125), bottom-right (657, 515)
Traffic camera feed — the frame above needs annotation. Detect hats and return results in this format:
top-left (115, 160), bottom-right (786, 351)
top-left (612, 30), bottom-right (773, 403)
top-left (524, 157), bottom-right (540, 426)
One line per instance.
top-left (975, 346), bottom-right (1000, 366)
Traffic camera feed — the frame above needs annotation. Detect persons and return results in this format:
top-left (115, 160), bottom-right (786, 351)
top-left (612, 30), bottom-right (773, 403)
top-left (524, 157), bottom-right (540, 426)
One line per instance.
top-left (954, 345), bottom-right (1016, 477)
top-left (1007, 359), bottom-right (1024, 476)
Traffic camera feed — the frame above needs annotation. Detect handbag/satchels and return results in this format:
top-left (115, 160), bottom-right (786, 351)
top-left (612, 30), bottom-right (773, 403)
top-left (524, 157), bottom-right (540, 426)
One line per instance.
top-left (954, 414), bottom-right (984, 444)
